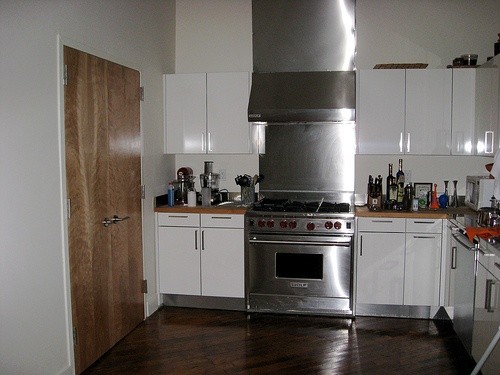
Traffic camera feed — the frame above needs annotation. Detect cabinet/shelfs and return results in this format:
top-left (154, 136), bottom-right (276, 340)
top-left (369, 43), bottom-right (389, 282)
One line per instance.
top-left (445, 232), bottom-right (477, 356)
top-left (357, 69), bottom-right (452, 154)
top-left (472, 240), bottom-right (500, 375)
top-left (353, 218), bottom-right (443, 306)
top-left (156, 211), bottom-right (245, 298)
top-left (453, 54), bottom-right (500, 156)
top-left (163, 71), bottom-right (252, 154)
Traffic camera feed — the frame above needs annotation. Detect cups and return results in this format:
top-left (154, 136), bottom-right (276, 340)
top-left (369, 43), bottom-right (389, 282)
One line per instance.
top-left (220, 193), bottom-right (230, 203)
top-left (240, 186), bottom-right (255, 205)
top-left (168, 185), bottom-right (175, 207)
top-left (201, 188), bottom-right (211, 208)
top-left (187, 191), bottom-right (196, 207)
top-left (412, 198), bottom-right (419, 212)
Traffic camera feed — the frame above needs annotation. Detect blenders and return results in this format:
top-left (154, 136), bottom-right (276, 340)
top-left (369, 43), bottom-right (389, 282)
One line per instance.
top-left (169, 167), bottom-right (195, 204)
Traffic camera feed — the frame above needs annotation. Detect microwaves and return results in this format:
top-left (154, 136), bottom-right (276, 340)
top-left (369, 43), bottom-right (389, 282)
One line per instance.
top-left (465, 175), bottom-right (495, 212)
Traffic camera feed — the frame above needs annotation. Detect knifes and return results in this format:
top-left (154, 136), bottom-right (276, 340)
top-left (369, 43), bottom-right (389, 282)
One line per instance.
top-left (367, 175), bottom-right (382, 198)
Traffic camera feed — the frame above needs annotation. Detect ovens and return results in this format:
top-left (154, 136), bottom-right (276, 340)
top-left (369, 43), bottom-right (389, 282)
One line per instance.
top-left (244, 234), bottom-right (354, 316)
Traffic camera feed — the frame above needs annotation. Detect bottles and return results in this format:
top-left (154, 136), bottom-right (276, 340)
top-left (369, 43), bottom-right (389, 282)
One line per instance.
top-left (386, 163), bottom-right (395, 200)
top-left (396, 158), bottom-right (404, 185)
top-left (397, 182), bottom-right (404, 210)
top-left (451, 180), bottom-right (459, 207)
top-left (388, 177), bottom-right (398, 209)
top-left (405, 183), bottom-right (414, 210)
top-left (444, 180), bottom-right (449, 207)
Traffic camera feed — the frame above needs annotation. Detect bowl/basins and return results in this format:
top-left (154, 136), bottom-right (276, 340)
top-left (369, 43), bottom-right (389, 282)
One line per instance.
top-left (169, 181), bottom-right (193, 201)
top-left (477, 207), bottom-right (500, 227)
top-left (463, 54), bottom-right (478, 65)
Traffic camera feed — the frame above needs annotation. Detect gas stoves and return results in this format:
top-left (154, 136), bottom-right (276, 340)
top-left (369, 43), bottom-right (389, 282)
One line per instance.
top-left (244, 198), bottom-right (355, 237)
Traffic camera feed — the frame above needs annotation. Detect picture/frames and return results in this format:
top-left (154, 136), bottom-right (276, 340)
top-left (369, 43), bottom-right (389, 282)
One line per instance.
top-left (414, 183), bottom-right (432, 203)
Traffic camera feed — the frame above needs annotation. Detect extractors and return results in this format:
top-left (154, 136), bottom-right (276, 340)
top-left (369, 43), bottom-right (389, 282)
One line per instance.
top-left (247, 71), bottom-right (357, 124)
